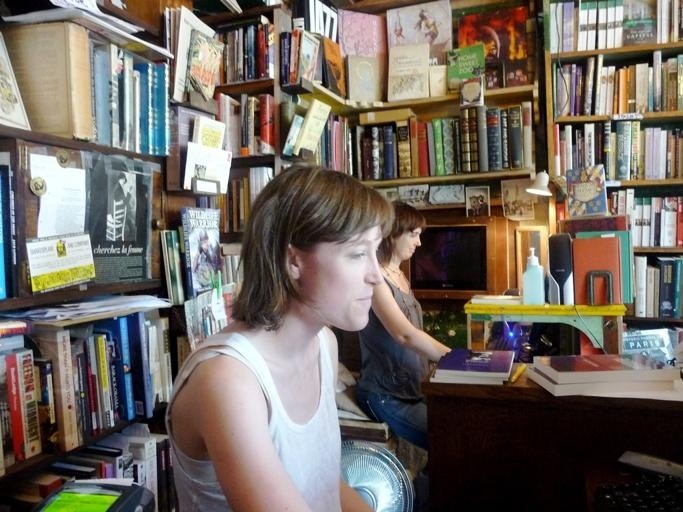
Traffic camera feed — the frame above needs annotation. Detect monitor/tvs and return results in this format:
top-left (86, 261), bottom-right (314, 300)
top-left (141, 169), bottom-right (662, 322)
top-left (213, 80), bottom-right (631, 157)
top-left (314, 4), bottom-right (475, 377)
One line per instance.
top-left (411, 226), bottom-right (486, 290)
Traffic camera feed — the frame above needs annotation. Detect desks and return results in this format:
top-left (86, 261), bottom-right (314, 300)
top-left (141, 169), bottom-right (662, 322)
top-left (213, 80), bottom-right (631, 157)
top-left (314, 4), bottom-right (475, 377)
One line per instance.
top-left (421, 354), bottom-right (681, 491)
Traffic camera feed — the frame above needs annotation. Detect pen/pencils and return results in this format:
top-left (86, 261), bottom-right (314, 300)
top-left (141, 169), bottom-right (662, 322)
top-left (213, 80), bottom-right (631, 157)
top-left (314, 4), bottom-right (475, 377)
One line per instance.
top-left (510, 363), bottom-right (527, 383)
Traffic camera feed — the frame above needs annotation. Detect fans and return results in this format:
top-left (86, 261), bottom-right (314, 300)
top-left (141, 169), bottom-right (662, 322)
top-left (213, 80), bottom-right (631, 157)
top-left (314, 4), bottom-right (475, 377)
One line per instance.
top-left (340, 439), bottom-right (414, 512)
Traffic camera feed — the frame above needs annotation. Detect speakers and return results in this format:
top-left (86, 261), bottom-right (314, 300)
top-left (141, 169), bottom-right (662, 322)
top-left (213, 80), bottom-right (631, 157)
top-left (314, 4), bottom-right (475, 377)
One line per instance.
top-left (547, 233), bottom-right (575, 306)
top-left (502, 289), bottom-right (521, 337)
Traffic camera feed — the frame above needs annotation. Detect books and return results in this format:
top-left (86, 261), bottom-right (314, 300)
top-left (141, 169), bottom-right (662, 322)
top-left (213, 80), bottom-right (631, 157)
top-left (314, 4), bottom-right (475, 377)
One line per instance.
top-left (427, 368), bottom-right (505, 388)
top-left (433, 348), bottom-right (515, 379)
top-left (532, 352), bottom-right (681, 385)
top-left (523, 366), bottom-right (681, 398)
top-left (458, 204), bottom-right (682, 349)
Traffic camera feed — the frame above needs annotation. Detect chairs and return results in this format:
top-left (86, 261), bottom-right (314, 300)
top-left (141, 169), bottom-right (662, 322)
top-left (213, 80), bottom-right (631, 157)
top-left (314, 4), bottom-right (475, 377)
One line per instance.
top-left (339, 371), bottom-right (389, 442)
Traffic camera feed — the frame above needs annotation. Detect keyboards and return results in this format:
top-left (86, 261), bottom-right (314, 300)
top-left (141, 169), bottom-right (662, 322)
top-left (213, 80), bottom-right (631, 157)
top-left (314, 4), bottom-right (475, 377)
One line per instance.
top-left (494, 336), bottom-right (523, 362)
top-left (597, 475), bottom-right (683, 512)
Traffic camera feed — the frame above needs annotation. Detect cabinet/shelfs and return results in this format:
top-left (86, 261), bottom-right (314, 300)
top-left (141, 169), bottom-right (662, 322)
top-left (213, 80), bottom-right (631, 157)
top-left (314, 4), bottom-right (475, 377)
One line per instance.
top-left (248, 0), bottom-right (540, 301)
top-left (544, 2), bottom-right (683, 322)
top-left (2, 3), bottom-right (247, 511)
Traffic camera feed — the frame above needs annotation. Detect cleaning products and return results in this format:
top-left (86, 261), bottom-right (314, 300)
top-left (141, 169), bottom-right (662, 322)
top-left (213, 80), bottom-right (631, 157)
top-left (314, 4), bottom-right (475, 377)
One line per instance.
top-left (522, 247), bottom-right (545, 306)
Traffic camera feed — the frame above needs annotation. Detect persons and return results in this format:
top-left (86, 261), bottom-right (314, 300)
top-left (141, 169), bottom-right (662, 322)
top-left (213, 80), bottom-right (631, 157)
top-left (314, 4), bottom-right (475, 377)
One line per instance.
top-left (163, 161), bottom-right (395, 512)
top-left (356, 198), bottom-right (454, 452)
